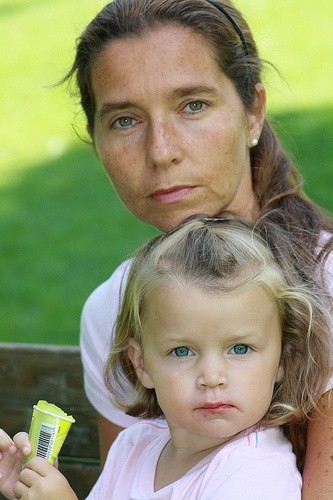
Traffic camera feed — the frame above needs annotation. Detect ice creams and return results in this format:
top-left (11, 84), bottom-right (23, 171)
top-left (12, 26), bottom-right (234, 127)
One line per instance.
top-left (22, 399), bottom-right (76, 473)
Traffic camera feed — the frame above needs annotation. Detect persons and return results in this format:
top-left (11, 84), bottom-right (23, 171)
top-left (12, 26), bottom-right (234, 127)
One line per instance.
top-left (51, 1), bottom-right (333, 500)
top-left (0, 208), bottom-right (333, 500)
